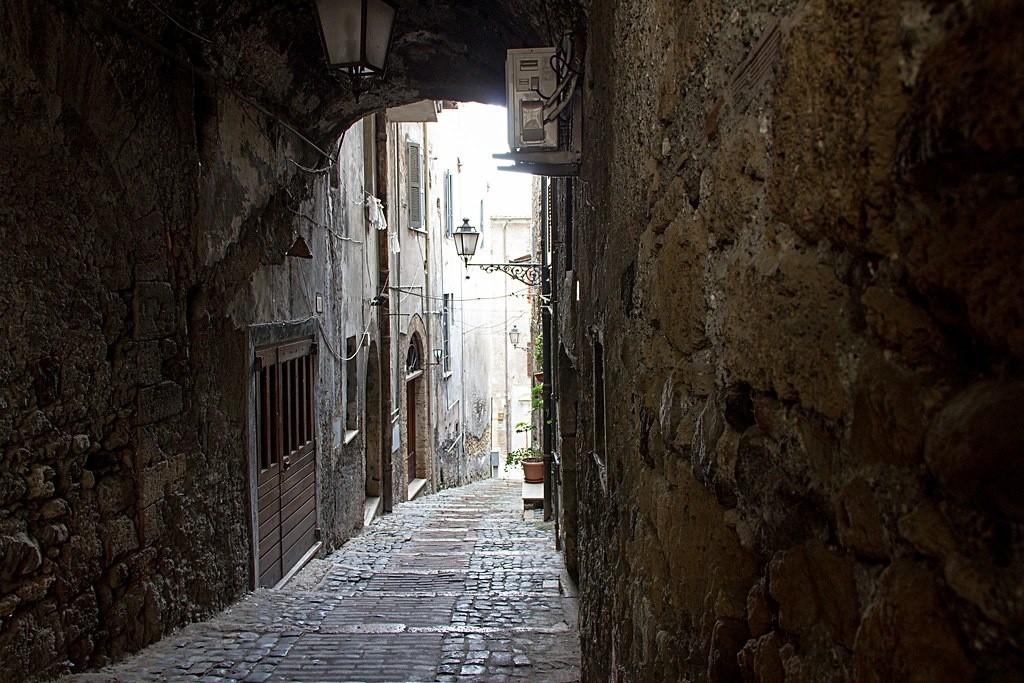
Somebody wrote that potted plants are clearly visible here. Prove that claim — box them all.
[532,331,543,381]
[504,420,545,483]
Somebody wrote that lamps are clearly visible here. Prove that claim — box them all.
[310,0,398,103]
[508,324,531,352]
[452,216,543,286]
[427,345,443,366]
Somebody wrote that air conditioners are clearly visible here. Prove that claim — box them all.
[504,47,561,150]
